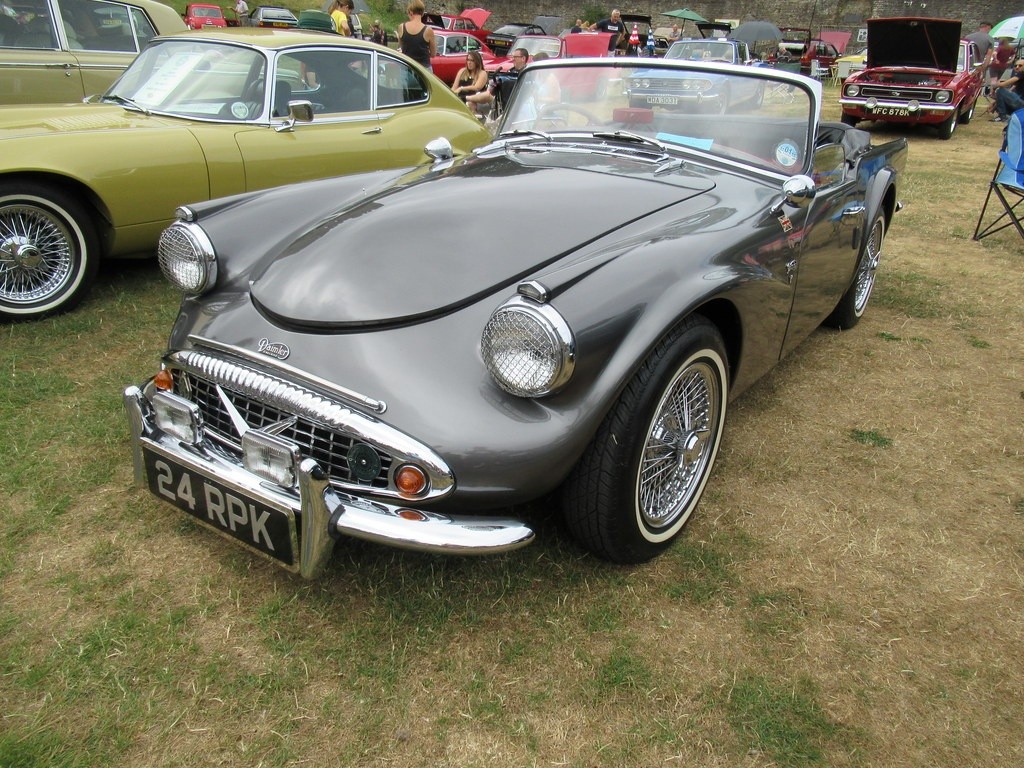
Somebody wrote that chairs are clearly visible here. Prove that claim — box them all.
[973,111,1024,243]
[236,77,291,119]
[763,60,854,104]
[692,49,703,59]
[0,14,20,47]
[14,16,83,49]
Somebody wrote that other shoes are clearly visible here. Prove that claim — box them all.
[993,116,1009,121]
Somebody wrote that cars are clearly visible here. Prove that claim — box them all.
[767,26,868,81]
[621,36,769,113]
[506,34,617,65]
[837,15,986,142]
[429,30,534,89]
[181,3,227,28]
[439,8,493,43]
[484,14,563,53]
[248,5,300,28]
[0,26,494,328]
[618,13,736,57]
[0,0,192,105]
[127,56,910,585]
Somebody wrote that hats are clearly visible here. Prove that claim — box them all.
[980,21,993,29]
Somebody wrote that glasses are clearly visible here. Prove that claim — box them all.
[513,55,523,59]
[466,59,474,62]
[1014,64,1023,68]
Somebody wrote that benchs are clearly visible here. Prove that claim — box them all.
[78,23,147,50]
[603,114,871,163]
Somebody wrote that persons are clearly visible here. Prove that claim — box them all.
[780,47,792,59]
[570,19,590,34]
[372,27,381,43]
[452,51,487,96]
[990,37,1015,97]
[327,0,354,36]
[668,24,681,47]
[965,21,994,67]
[227,0,249,27]
[460,48,529,112]
[398,0,436,73]
[590,9,626,57]
[989,59,1024,121]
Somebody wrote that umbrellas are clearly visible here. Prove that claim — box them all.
[661,8,709,36]
[730,21,783,51]
[988,16,1024,52]
[629,25,639,44]
[646,27,655,50]
